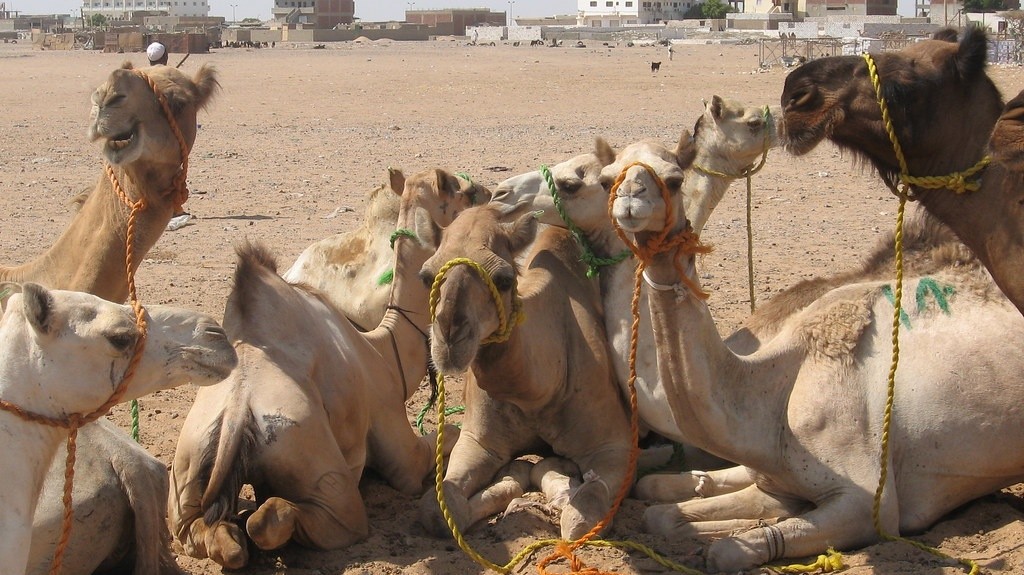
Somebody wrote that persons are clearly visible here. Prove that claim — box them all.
[148,41,168,67]
[668,46,675,61]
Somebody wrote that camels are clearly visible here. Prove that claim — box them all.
[0,16,1024,574]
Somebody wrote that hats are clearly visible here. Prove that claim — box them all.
[147,42,165,62]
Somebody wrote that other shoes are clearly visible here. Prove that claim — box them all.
[173,211,195,220]
